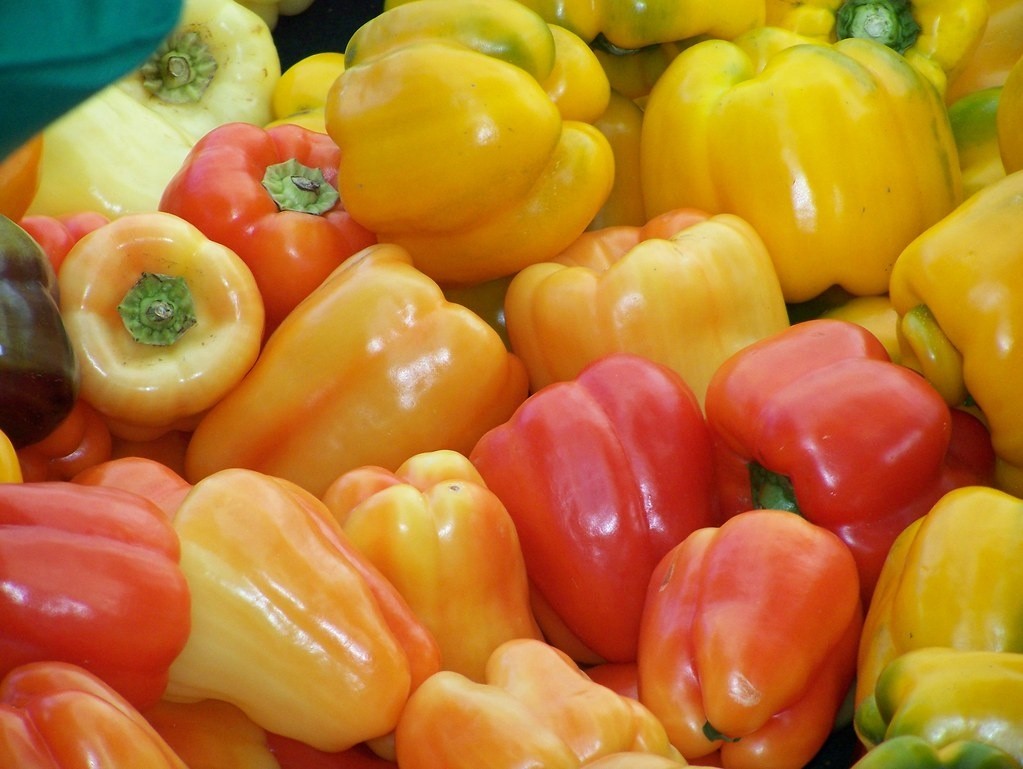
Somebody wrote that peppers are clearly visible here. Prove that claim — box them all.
[0,0,1023,769]
